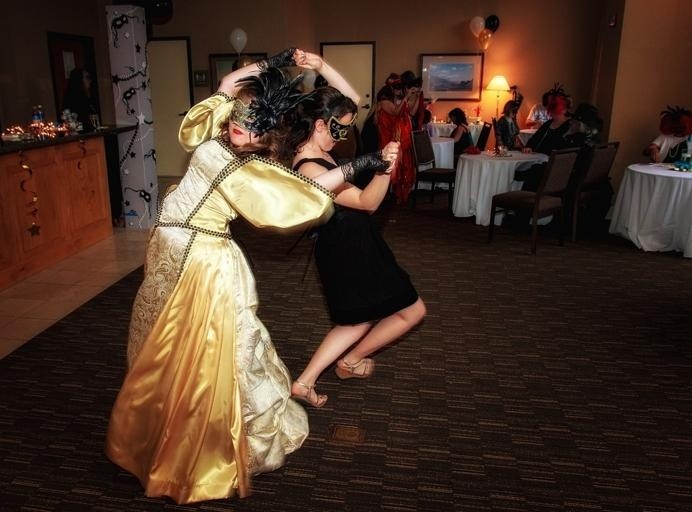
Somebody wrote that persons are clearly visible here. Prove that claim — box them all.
[359,71,427,212]
[494,101,524,150]
[286,49,429,406]
[63,68,100,115]
[522,90,580,156]
[525,98,553,128]
[642,106,691,165]
[514,102,604,231]
[448,108,474,155]
[101,47,398,508]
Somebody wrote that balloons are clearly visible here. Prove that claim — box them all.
[469,15,486,38]
[478,28,494,50]
[485,14,499,32]
[147,1,173,18]
[228,26,248,54]
[237,54,253,69]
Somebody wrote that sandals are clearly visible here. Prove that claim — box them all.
[291,382,328,408]
[334,360,377,380]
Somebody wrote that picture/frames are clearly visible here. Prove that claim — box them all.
[418,52,485,102]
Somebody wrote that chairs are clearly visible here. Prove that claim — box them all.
[351,118,620,255]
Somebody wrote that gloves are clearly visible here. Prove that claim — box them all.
[340,148,391,182]
[255,47,298,72]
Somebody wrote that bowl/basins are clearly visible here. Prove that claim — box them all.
[54,129,66,136]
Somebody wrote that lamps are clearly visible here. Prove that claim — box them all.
[485,73,511,118]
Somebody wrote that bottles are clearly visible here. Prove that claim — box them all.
[31,104,44,123]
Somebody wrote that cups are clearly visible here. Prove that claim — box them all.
[486,143,511,157]
[522,147,532,156]
[432,115,436,123]
[89,114,100,129]
[446,112,453,125]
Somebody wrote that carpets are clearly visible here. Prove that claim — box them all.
[0,189,692,510]
